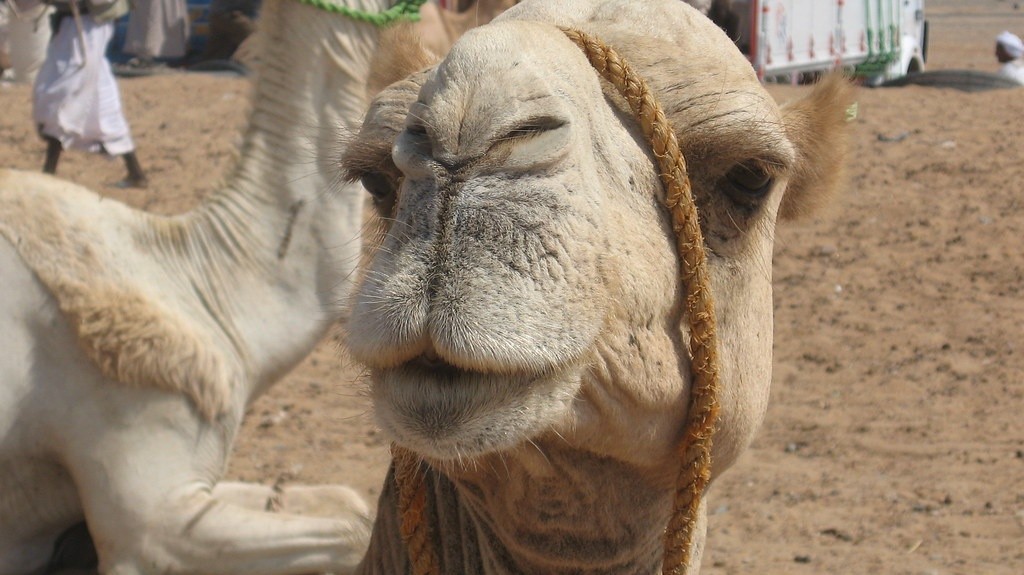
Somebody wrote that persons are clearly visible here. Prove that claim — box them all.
[30,0,149,189]
[995,30,1024,85]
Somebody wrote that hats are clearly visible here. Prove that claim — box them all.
[997,31,1024,59]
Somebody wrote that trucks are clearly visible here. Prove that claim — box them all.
[715,0,930,83]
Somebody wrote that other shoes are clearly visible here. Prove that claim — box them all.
[127,55,153,69]
